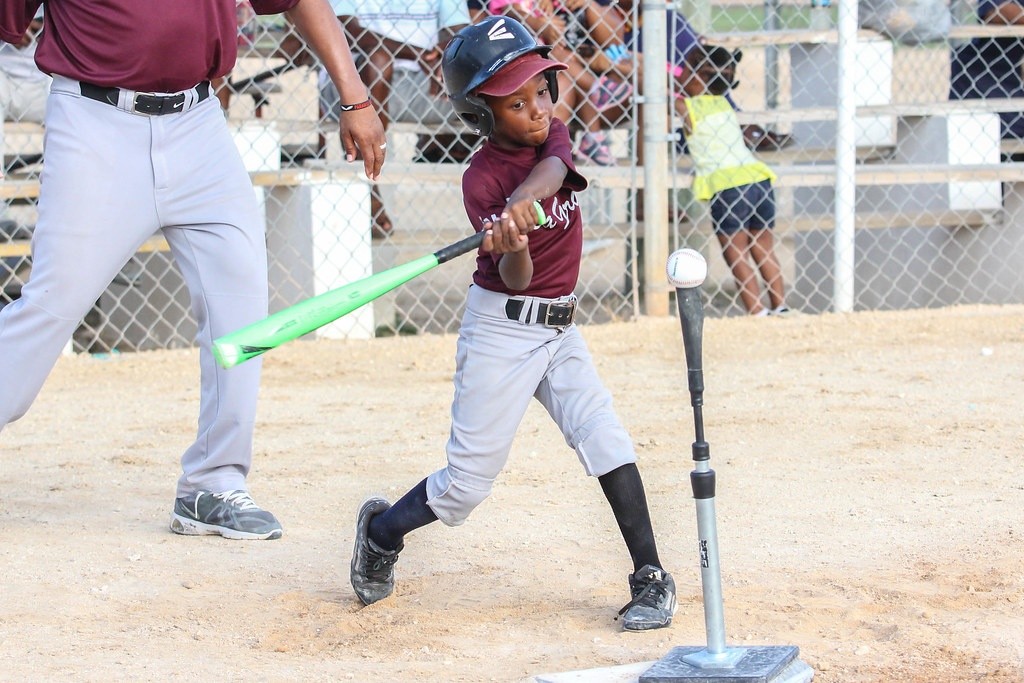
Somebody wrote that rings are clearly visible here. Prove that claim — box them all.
[379,142,386,148]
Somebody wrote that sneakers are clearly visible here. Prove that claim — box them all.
[170,489,284,540]
[574,134,617,165]
[593,73,629,111]
[350,496,405,605]
[614,564,678,632]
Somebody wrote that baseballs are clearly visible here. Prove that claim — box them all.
[665,248,707,288]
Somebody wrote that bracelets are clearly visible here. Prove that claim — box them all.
[340,96,371,110]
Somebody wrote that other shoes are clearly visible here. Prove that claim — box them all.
[748,127,794,149]
[372,194,395,239]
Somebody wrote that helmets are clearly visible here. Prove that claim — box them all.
[442,15,560,136]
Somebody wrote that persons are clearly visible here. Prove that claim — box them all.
[274,0,794,168]
[636,44,786,317]
[0,0,386,538]
[352,14,679,631]
[973,0,1023,84]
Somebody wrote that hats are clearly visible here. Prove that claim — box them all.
[473,51,569,95]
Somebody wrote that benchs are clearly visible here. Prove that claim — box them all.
[0,0,1024,257]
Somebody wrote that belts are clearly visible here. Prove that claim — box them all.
[506,298,578,328]
[80,80,209,116]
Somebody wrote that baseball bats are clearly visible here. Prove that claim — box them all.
[208,200,548,371]
